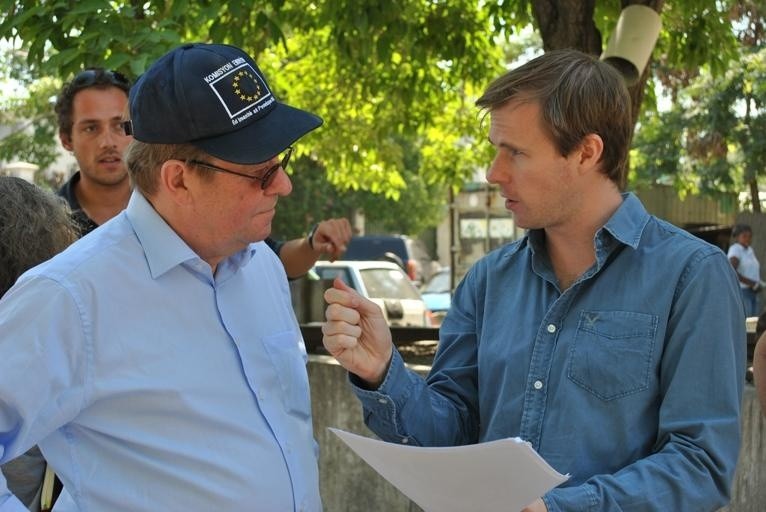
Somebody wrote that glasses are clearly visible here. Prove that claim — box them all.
[73,69,122,87]
[180,146,296,190]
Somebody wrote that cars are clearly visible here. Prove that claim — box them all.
[310,261,450,326]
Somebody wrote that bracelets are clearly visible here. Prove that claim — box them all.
[308,223,319,249]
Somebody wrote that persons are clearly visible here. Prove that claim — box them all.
[1,177,83,511]
[322,50,746,512]
[727,224,766,317]
[55,67,351,282]
[2,43,323,511]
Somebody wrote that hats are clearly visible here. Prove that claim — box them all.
[124,42,324,163]
